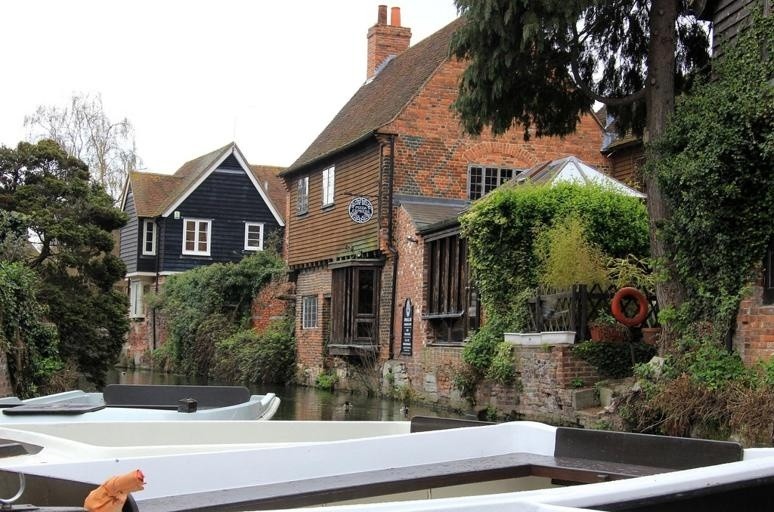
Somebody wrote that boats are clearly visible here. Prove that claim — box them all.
[3,386,282,422]
[3,415,774,511]
